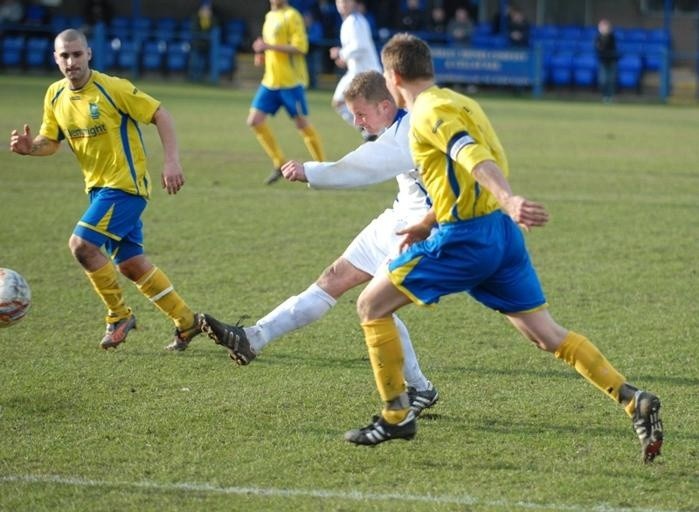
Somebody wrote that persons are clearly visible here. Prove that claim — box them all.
[316,0,530,96]
[1,1,217,60]
[594,19,620,98]
[246,0,326,188]
[342,29,662,465]
[329,0,386,142]
[192,72,440,419]
[9,28,204,353]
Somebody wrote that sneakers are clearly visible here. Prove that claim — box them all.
[200,311,256,366]
[344,380,439,446]
[164,312,202,350]
[262,165,282,186]
[628,389,663,465]
[100,314,137,349]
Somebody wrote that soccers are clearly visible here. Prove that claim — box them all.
[0,267,33,328]
